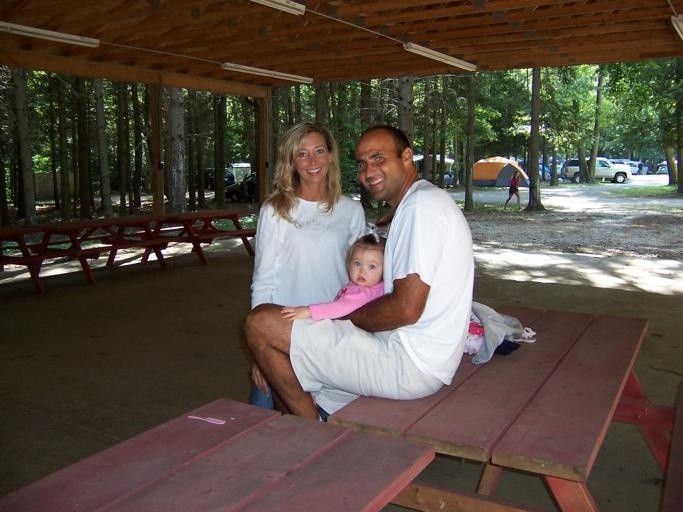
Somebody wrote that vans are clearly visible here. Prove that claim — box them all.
[202,169,234,191]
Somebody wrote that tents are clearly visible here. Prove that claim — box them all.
[518,158,552,182]
[471,155,530,187]
[411,153,459,186]
[655,159,681,174]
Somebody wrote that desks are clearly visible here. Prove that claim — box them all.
[0,208,255,295]
[327,306,683,512]
[0,397,537,512]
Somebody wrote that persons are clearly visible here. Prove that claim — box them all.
[241,124,475,421]
[279,232,386,322]
[503,170,521,211]
[247,121,366,412]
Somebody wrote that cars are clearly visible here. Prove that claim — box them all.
[611,158,643,175]
[655,165,668,174]
[517,158,566,179]
[224,171,258,201]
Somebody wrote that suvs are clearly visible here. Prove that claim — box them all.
[562,156,631,183]
